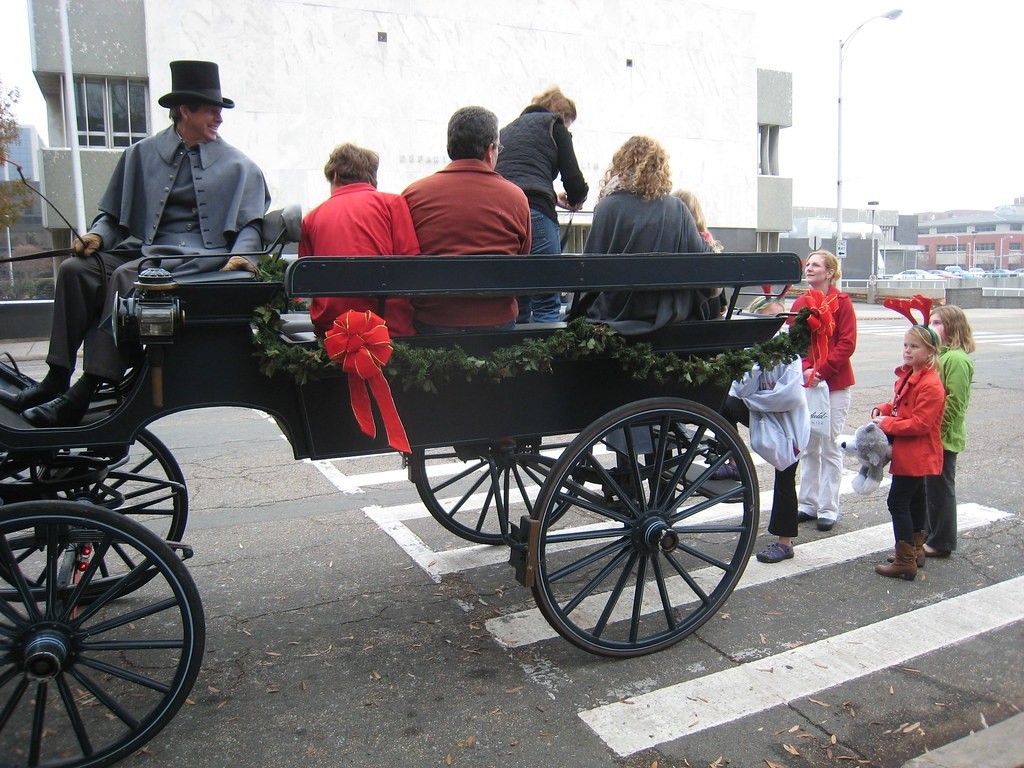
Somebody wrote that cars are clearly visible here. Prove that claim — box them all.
[895,269,934,280]
[928,265,1024,279]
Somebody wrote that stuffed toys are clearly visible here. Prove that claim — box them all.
[835,423,892,495]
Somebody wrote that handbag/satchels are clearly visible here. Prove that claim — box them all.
[806,378,831,440]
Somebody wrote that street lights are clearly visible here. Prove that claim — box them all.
[945,234,958,266]
[1000,236,1014,268]
[867,201,879,275]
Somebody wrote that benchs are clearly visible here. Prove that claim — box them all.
[283,252,803,360]
[132,203,302,327]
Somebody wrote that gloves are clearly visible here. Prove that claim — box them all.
[74,233,101,256]
[220,255,261,279]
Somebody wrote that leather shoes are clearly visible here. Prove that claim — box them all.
[0,384,60,413]
[23,393,83,427]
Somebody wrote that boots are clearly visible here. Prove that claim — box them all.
[887,530,927,567]
[875,540,918,581]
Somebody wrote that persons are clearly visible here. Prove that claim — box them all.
[786,252,857,529]
[709,285,810,563]
[296,142,422,337]
[871,295,946,579]
[669,188,728,320]
[15,60,272,425]
[922,305,976,556]
[402,106,532,334]
[606,422,675,503]
[494,85,589,322]
[560,137,717,336]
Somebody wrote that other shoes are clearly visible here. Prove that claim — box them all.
[921,545,951,557]
[817,518,835,531]
[798,510,816,523]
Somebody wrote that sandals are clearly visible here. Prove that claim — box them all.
[756,540,794,563]
[710,463,741,481]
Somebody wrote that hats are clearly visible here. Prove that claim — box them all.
[158,60,236,108]
